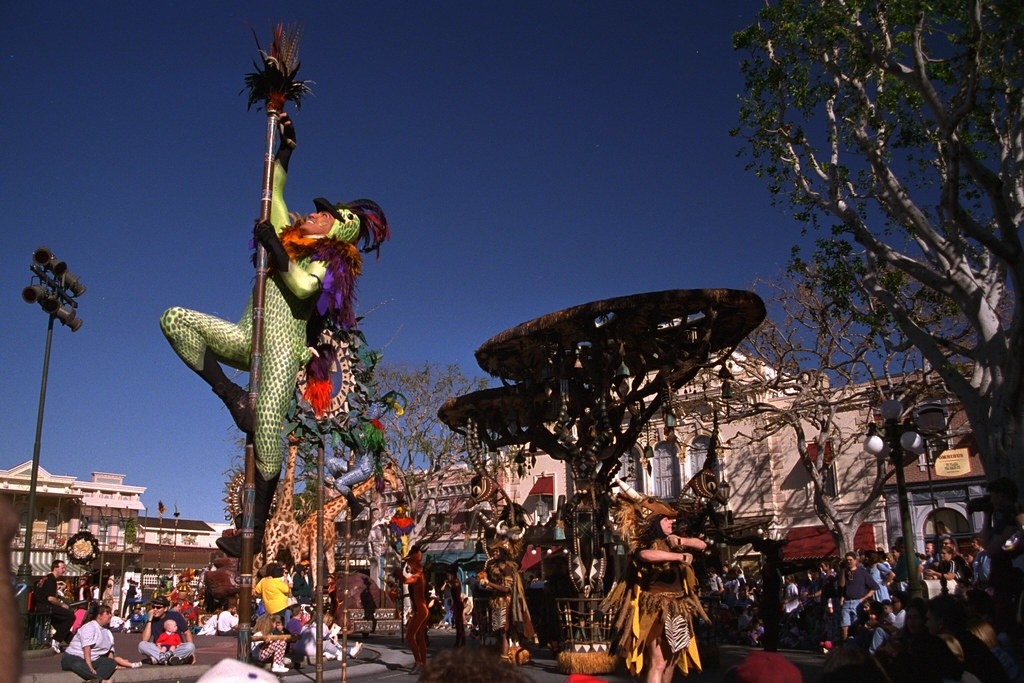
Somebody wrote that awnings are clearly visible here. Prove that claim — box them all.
[807,441,831,463]
[769,523,876,561]
[529,476,553,496]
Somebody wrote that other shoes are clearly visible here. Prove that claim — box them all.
[135,661,142,669]
[159,653,168,662]
[324,653,334,661]
[150,655,158,665]
[169,657,184,665]
[337,652,344,661]
[216,529,262,556]
[211,383,248,425]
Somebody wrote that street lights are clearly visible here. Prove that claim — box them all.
[13,246,86,644]
[863,400,923,600]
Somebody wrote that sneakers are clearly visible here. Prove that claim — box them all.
[272,663,289,673]
[51,638,61,653]
[282,658,291,665]
[350,642,363,658]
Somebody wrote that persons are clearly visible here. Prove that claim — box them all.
[0,106,1024,683]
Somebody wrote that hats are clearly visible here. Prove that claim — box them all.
[152,596,170,606]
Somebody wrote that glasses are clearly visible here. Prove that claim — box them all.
[153,605,166,609]
